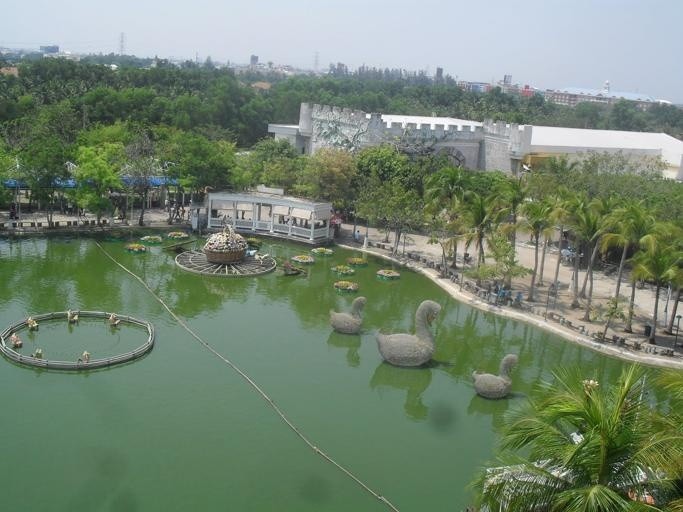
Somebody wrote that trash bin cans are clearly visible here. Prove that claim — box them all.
[644,325,651,336]
[12,223,17,227]
[212,209,217,218]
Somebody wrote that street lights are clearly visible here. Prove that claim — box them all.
[7,158,26,211]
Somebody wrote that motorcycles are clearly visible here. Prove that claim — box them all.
[559,246,583,270]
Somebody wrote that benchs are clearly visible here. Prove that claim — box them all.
[3,217,124,228]
